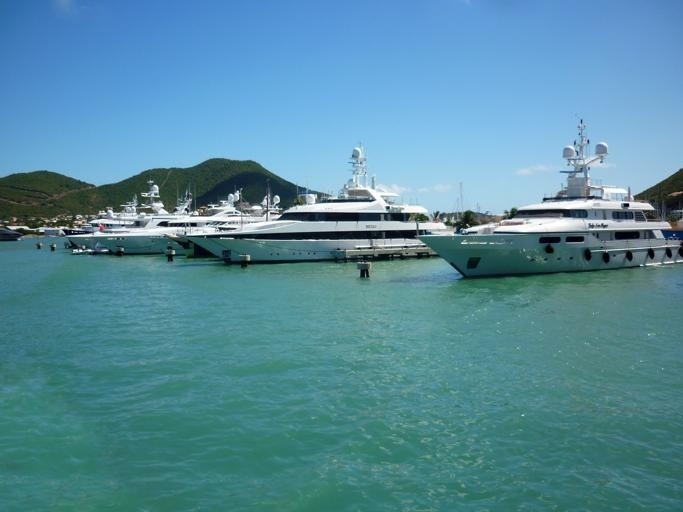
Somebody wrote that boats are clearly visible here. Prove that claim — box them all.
[1,175,244,242]
[176,137,454,265]
[415,118,683,279]
[64,179,317,257]
